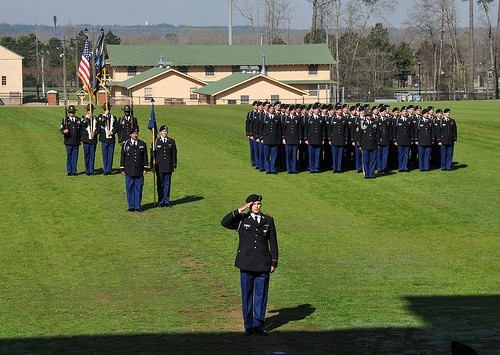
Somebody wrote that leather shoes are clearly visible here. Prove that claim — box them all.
[254,326,268,336]
[244,329,253,335]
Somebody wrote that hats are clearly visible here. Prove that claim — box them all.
[252,100,450,114]
[364,108,372,116]
[129,128,139,134]
[246,194,262,203]
[159,125,168,132]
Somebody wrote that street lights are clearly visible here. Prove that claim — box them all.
[418,59,422,95]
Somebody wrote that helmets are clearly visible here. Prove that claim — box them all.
[85,103,96,109]
[121,105,131,111]
[101,102,112,108]
[66,105,78,111]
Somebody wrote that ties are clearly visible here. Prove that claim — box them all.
[133,141,136,146]
[164,139,165,143]
[270,115,451,124]
[254,216,258,224]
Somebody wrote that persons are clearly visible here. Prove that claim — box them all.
[118,105,139,147]
[120,128,148,212]
[80,103,100,174]
[221,194,278,337]
[60,106,82,176]
[96,102,119,174]
[245,100,457,179]
[149,125,177,207]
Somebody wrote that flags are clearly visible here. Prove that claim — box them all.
[147,104,158,139]
[78,37,108,94]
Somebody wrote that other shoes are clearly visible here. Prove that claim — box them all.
[164,203,169,207]
[104,172,112,175]
[364,177,375,180]
[251,163,451,174]
[136,209,141,212]
[73,173,78,176]
[127,208,135,211]
[66,173,71,176]
[158,204,164,207]
[86,173,93,176]
